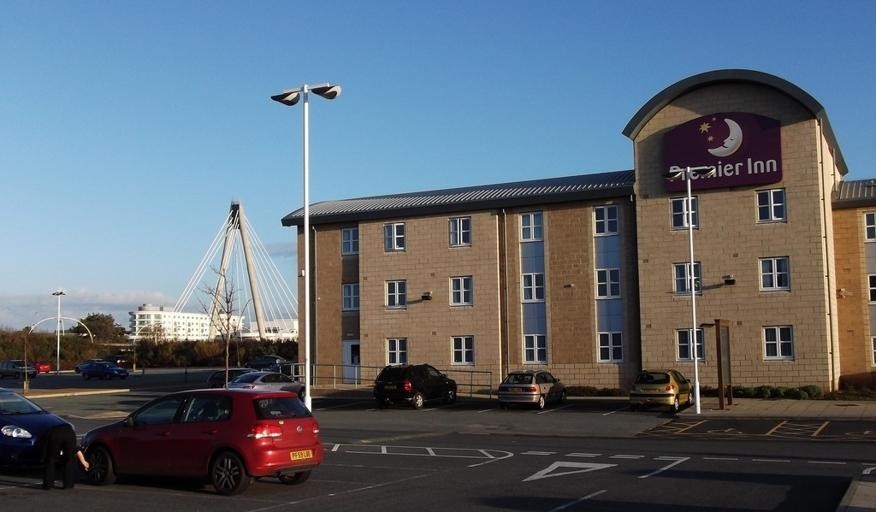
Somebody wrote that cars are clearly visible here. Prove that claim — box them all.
[0,357,39,378]
[625,364,695,413]
[81,361,132,379]
[0,385,82,473]
[71,358,104,374]
[207,368,259,388]
[243,353,287,369]
[496,368,570,410]
[222,370,305,399]
[77,385,329,497]
[32,359,52,374]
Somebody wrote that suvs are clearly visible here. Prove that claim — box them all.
[369,363,459,412]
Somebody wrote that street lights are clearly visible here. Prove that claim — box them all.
[265,79,347,412]
[661,164,721,413]
[51,290,66,374]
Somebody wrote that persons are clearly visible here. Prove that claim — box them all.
[39,423,91,491]
